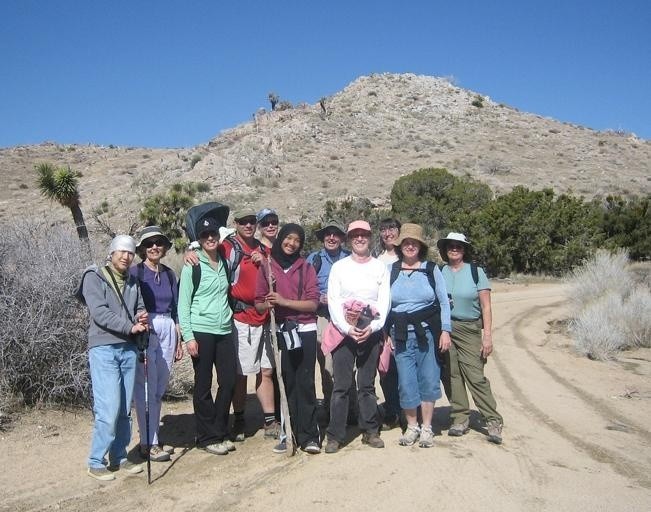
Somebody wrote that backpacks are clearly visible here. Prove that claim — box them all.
[176,255,229,342]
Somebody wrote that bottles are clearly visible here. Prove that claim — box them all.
[283,318,304,352]
[353,304,375,331]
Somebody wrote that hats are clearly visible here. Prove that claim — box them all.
[233,206,259,222]
[135,225,173,260]
[256,207,279,224]
[392,222,430,249]
[347,219,372,237]
[194,215,220,239]
[315,217,348,245]
[436,231,474,253]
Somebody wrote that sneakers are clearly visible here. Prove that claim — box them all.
[231,422,244,441]
[222,441,236,451]
[264,420,282,440]
[419,426,434,447]
[157,443,174,453]
[140,444,170,461]
[399,426,420,445]
[88,468,115,480]
[205,443,228,455]
[448,420,470,436]
[111,460,143,474]
[487,421,503,444]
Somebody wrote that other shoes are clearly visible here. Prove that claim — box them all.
[362,433,384,448]
[381,414,398,429]
[273,439,287,453]
[305,441,321,453]
[325,439,341,453]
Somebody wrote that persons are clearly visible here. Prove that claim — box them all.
[72,235,150,481]
[437,231,504,444]
[129,225,184,461]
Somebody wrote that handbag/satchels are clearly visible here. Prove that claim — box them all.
[276,322,304,354]
[228,295,248,314]
[134,332,148,351]
